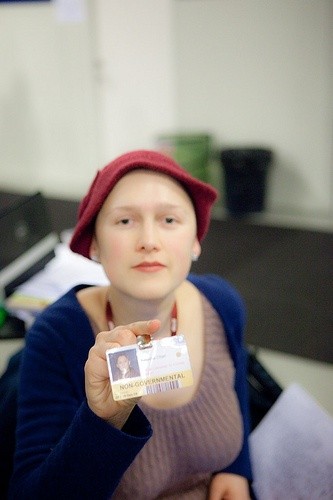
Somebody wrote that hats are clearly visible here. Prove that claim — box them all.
[68,152,219,265]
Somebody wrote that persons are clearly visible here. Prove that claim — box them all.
[13,149,256,500]
[116,355,137,380]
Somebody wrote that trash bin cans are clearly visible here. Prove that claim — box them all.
[222,148,272,213]
[163,132,212,184]
